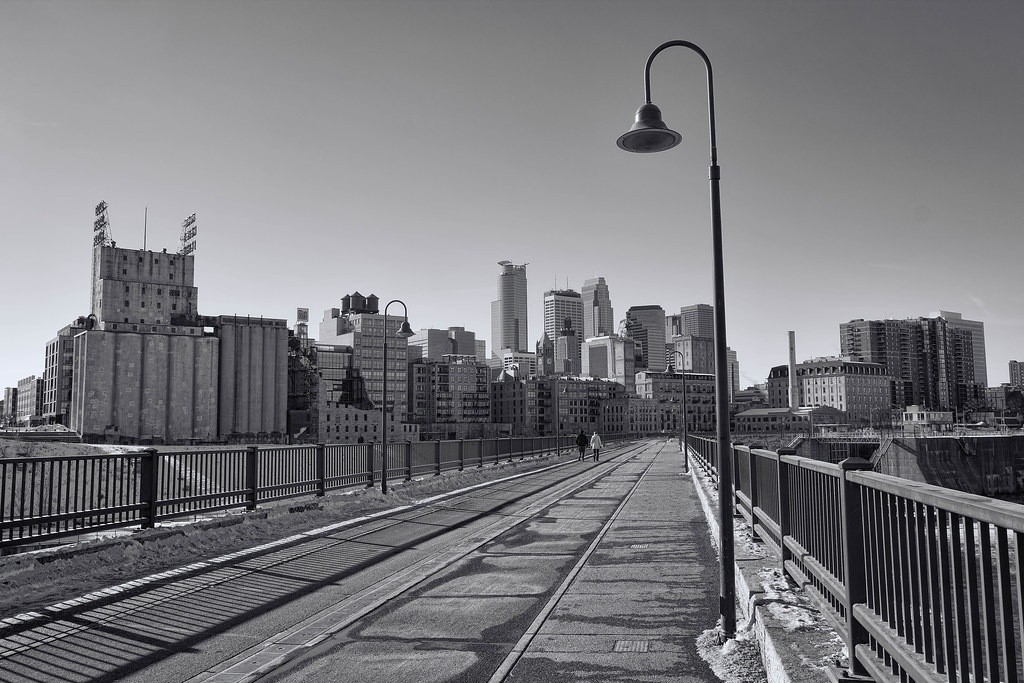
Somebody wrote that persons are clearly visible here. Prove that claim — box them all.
[590,431,602,462]
[575,430,588,461]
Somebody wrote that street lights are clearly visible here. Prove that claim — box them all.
[615,39,737,645]
[665,351,689,473]
[381,300,416,495]
[670,396,682,452]
[556,378,568,457]
